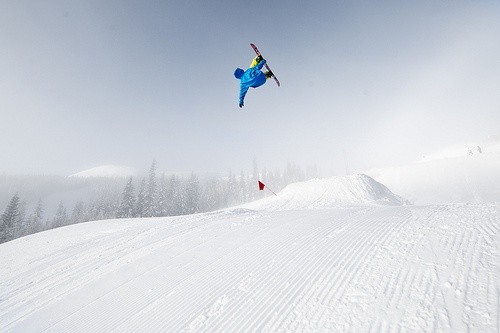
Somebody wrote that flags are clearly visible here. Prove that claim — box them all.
[258,181,265,190]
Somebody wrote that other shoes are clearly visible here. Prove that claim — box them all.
[265,71,273,77]
[255,56,261,63]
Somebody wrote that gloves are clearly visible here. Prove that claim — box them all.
[239,104,244,108]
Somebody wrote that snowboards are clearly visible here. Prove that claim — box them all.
[249,42,281,88]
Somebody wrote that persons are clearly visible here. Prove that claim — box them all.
[234,54,273,108]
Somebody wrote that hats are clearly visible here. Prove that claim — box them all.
[234,68,245,79]
